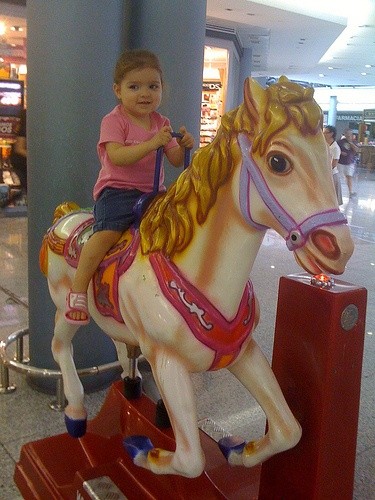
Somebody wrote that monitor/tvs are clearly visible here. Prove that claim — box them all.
[0,79,24,116]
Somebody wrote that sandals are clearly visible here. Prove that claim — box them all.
[65,289,89,324]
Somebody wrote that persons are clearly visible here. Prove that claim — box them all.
[64,50,195,325]
[324,126,369,208]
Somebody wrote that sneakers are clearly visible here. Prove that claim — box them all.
[350,192,357,199]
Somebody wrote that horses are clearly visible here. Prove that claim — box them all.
[39,74,354,480]
